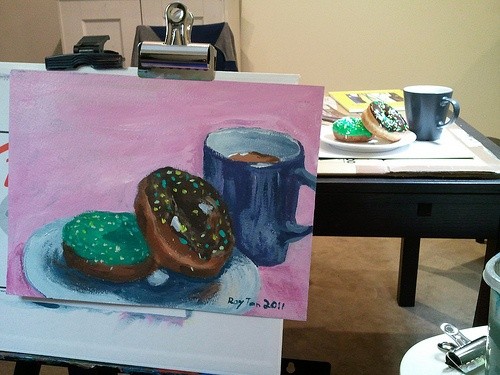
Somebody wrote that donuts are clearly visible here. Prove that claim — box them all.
[332,117,374,142]
[361,100,407,143]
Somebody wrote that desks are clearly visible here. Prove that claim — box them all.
[311,107,500,327]
[399,325,489,375]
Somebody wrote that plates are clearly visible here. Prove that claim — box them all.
[319,125,416,152]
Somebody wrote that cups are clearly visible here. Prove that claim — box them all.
[403,85,460,141]
[483,253,500,375]
